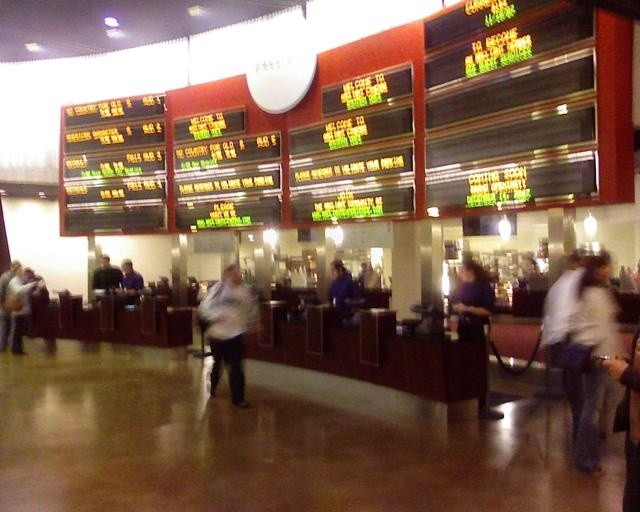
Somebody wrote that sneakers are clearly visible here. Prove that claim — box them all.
[573,460,601,473]
[231,398,248,407]
[478,410,504,420]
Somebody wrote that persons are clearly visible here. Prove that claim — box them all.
[448,256,504,419]
[540,247,640,512]
[92,255,144,305]
[196,263,262,409]
[0,256,57,357]
[327,258,364,317]
[359,262,379,288]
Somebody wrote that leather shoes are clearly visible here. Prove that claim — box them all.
[0,347,28,355]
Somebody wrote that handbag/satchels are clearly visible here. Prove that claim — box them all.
[198,319,208,333]
